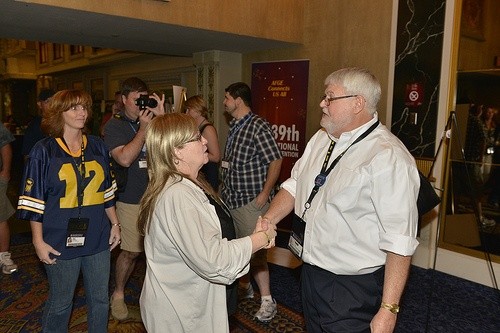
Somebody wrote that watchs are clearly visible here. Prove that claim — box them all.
[381,301,400,314]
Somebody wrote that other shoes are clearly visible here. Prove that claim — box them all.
[110,296,128,320]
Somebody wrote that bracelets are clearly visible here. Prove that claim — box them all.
[258,230,271,247]
[111,222,121,228]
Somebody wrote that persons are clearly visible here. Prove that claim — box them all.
[137,112,280,333]
[253,67,419,333]
[454,99,500,229]
[220,83,284,324]
[182,96,223,194]
[16,87,125,333]
[1,90,19,275]
[99,91,124,136]
[105,78,170,322]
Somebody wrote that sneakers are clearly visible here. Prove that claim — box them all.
[0,252,18,274]
[228,284,254,299]
[253,298,277,324]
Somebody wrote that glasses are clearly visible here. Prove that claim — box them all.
[320,95,366,106]
[186,134,201,143]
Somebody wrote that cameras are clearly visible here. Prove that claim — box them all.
[136,95,158,110]
